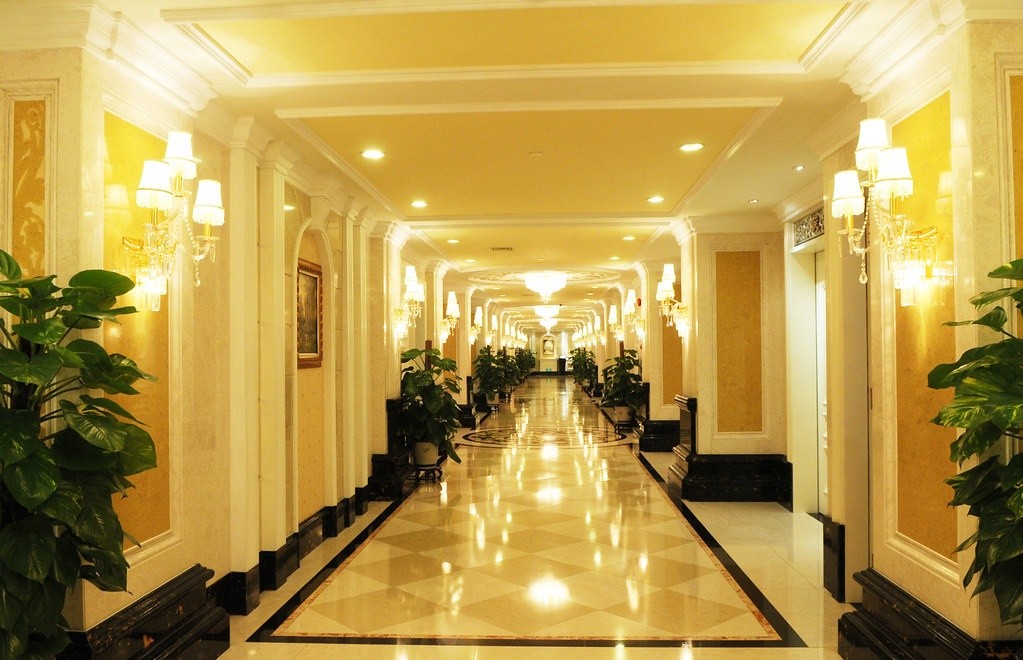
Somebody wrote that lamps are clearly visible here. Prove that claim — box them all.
[124,131,225,311]
[439,291,460,348]
[572,316,606,348]
[469,305,484,346]
[394,264,424,343]
[623,289,645,332]
[656,264,690,327]
[831,118,912,285]
[608,305,624,340]
[486,315,529,349]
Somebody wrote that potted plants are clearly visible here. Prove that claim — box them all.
[598,348,644,421]
[473,346,540,405]
[400,348,463,470]
[569,347,596,386]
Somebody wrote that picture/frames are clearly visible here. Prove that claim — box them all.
[297,258,323,369]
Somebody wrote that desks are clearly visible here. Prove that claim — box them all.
[556,359,566,376]
